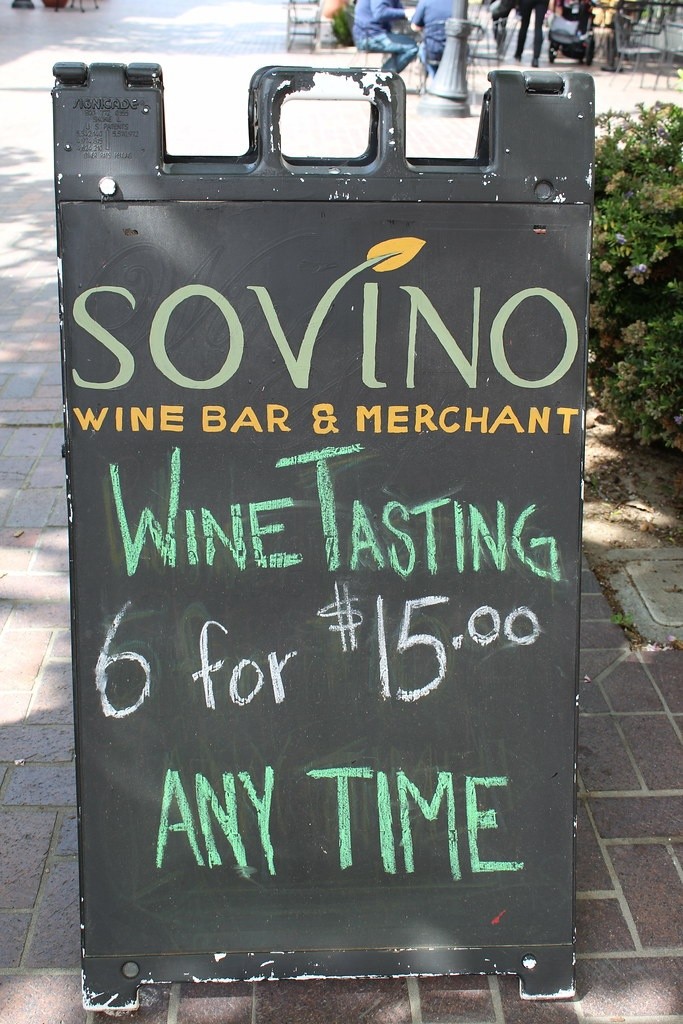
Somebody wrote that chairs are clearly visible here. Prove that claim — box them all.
[286,0,683,107]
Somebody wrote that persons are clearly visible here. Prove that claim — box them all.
[411,0,454,78]
[352,0,419,75]
[489,0,651,73]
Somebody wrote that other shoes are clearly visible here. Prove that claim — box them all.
[532,57,539,67]
[514,52,521,61]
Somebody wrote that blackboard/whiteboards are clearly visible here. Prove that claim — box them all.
[54,72,601,1010]
